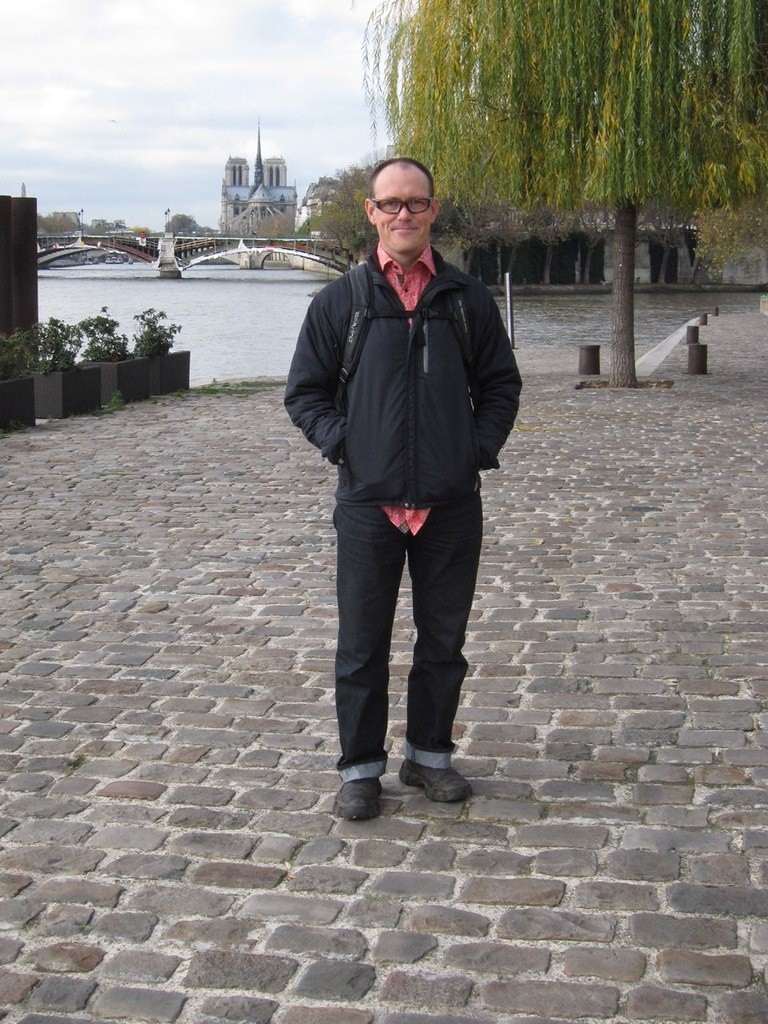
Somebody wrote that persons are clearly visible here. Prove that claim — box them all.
[284,157,523,820]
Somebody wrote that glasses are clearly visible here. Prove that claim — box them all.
[371,198,433,214]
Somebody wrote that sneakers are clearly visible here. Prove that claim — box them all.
[333,777,382,819]
[399,757,470,802]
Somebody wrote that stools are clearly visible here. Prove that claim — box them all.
[687,326,698,345]
[712,306,719,316]
[699,312,707,325]
[688,344,708,375]
[577,343,603,376]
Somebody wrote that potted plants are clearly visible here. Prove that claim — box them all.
[0,326,46,433]
[74,306,151,406]
[132,308,190,396]
[23,317,102,420]
